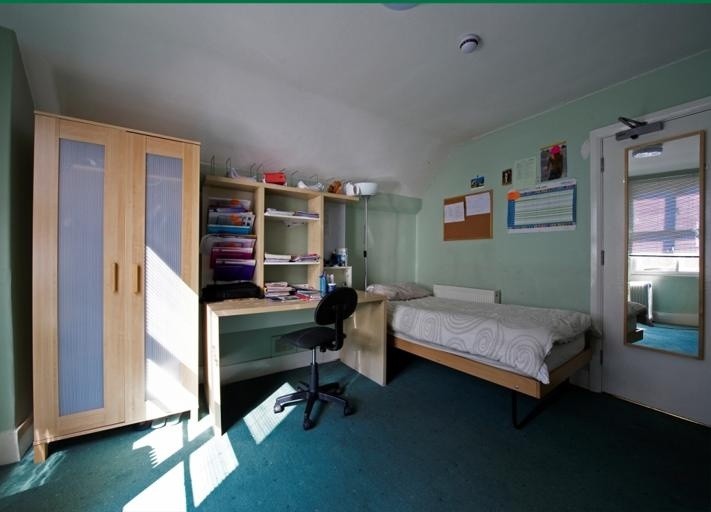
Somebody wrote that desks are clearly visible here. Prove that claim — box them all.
[203,289,388,435]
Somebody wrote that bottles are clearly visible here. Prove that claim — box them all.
[316,270,337,300]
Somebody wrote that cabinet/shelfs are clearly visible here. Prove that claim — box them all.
[30,110,203,464]
[202,175,360,295]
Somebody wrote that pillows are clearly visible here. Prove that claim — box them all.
[365,283,433,300]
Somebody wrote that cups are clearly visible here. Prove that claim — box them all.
[344,180,360,196]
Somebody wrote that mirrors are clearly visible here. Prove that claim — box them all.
[622,130,706,362]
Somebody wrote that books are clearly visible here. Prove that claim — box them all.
[201,196,324,304]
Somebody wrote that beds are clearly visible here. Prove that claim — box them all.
[386,296,593,429]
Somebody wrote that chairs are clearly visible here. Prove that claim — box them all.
[273,287,358,430]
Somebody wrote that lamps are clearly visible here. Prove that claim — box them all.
[355,182,378,291]
[631,143,663,160]
